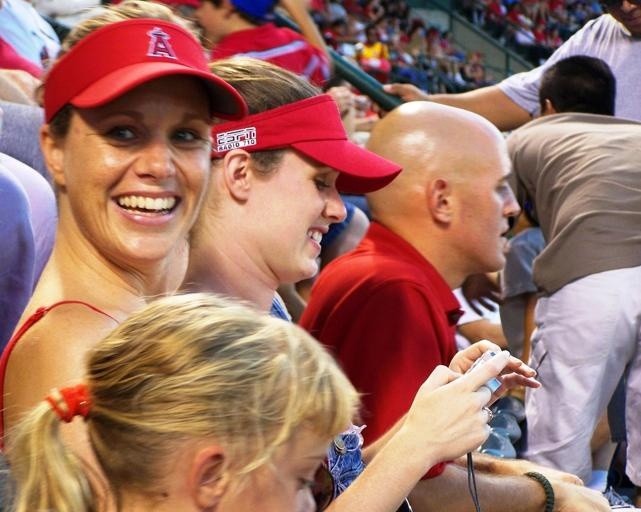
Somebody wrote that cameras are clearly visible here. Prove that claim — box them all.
[463,348,509,410]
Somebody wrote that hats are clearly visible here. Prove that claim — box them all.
[44,18,249,126]
[211,95,403,196]
[233,0,280,17]
